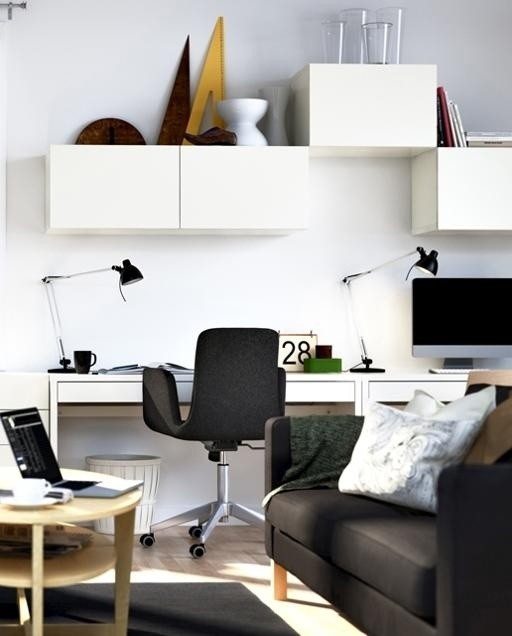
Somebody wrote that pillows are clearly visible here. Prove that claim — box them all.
[280,410,363,491]
[406,386,496,462]
[334,396,483,516]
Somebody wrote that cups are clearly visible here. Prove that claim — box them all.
[335,5,368,64]
[371,5,405,61]
[10,478,51,500]
[358,20,397,64]
[73,350,97,374]
[318,19,345,64]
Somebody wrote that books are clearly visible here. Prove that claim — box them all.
[98,361,194,374]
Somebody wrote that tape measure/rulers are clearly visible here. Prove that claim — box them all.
[182,17,226,145]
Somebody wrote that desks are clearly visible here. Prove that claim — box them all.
[0,372,510,541]
[2,462,141,635]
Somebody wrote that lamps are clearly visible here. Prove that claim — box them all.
[340,244,444,373]
[42,257,151,374]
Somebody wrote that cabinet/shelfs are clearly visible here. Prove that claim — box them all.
[46,144,309,233]
[409,146,510,236]
[288,58,438,148]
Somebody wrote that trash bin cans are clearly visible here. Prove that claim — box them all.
[86,454,162,535]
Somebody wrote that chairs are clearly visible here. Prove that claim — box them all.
[141,328,292,559]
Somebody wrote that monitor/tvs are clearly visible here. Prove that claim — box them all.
[412,278,512,369]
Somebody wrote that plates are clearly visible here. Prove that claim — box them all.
[2,496,57,508]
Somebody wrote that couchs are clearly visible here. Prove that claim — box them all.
[265,383,510,634]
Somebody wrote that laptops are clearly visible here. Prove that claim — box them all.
[0,406,144,498]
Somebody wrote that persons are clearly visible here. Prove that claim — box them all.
[437,85,512,146]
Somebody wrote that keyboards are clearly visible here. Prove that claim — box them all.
[429,369,490,374]
